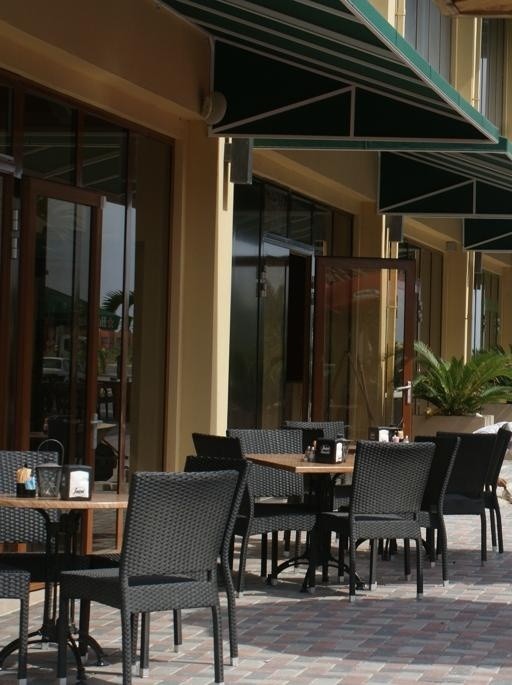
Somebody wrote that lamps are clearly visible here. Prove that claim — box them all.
[36,440,64,499]
[224,138,256,186]
[385,216,404,243]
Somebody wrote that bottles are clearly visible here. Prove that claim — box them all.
[305,446,315,461]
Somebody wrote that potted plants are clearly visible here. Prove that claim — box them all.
[411,338,510,438]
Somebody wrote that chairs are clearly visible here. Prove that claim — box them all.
[2,568,33,685]
[131,456,252,678]
[58,472,235,685]
[191,432,317,594]
[279,420,510,567]
[323,442,437,602]
[227,426,307,580]
[2,447,73,637]
[350,437,458,588]
